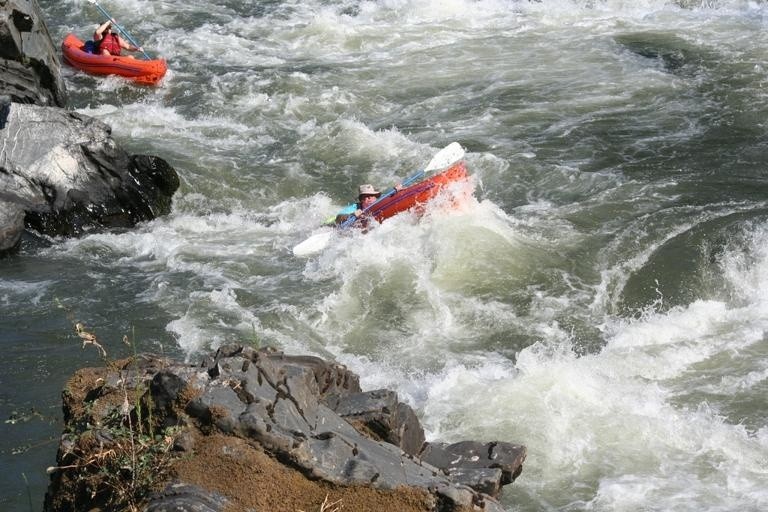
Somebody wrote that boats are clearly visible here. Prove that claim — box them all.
[323,163,467,235]
[62,32,168,84]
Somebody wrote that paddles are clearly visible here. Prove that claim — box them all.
[293,141,465,260]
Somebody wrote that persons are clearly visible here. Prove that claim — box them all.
[94,18,147,60]
[85,29,121,54]
[316,183,407,228]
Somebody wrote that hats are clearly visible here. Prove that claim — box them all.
[358,183,382,202]
